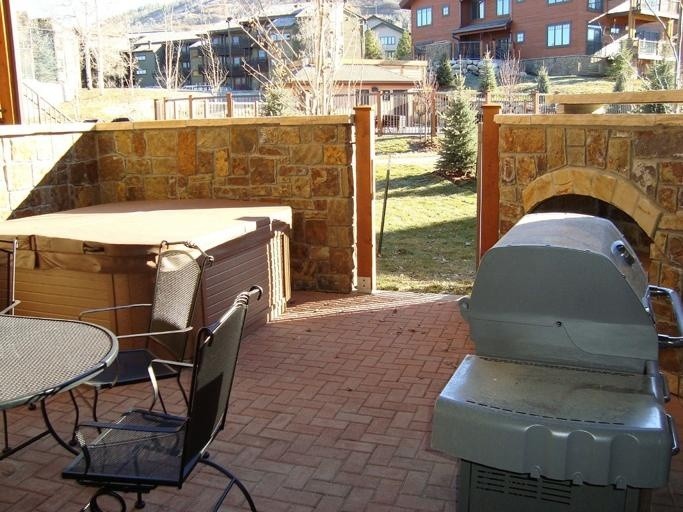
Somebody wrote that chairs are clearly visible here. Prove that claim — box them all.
[0,240,262,512]
